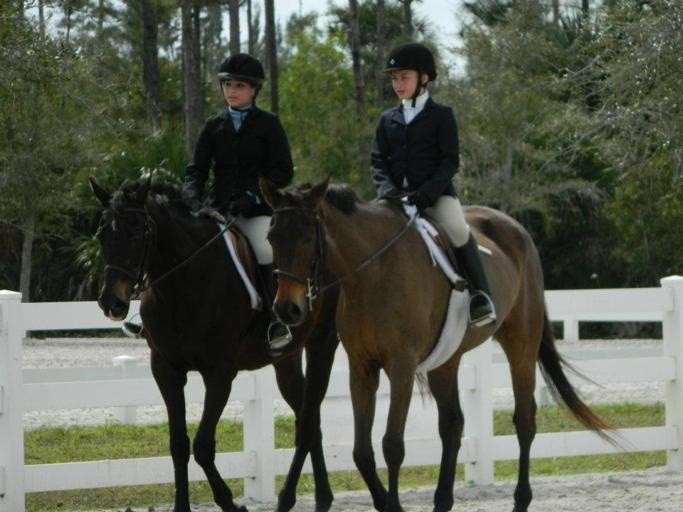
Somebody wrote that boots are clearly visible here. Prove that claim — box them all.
[453,230,491,316]
[258,261,288,340]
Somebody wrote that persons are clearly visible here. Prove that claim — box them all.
[366,44,498,327]
[120,52,293,360]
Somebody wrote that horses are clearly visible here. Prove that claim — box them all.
[88,171,339,512]
[258,171,641,512]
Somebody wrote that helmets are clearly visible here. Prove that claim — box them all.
[218,53,264,85]
[384,43,437,81]
